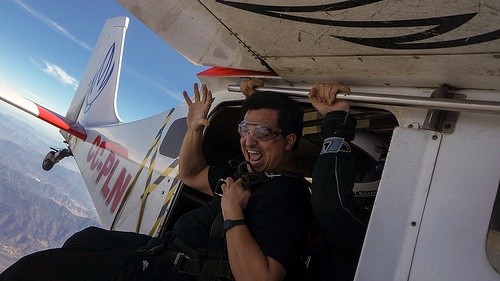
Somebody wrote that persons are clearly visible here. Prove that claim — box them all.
[241,76,387,281]
[0,81,310,281]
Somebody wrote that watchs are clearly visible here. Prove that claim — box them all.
[223,218,247,235]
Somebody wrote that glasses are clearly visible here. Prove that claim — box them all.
[238,120,285,140]
